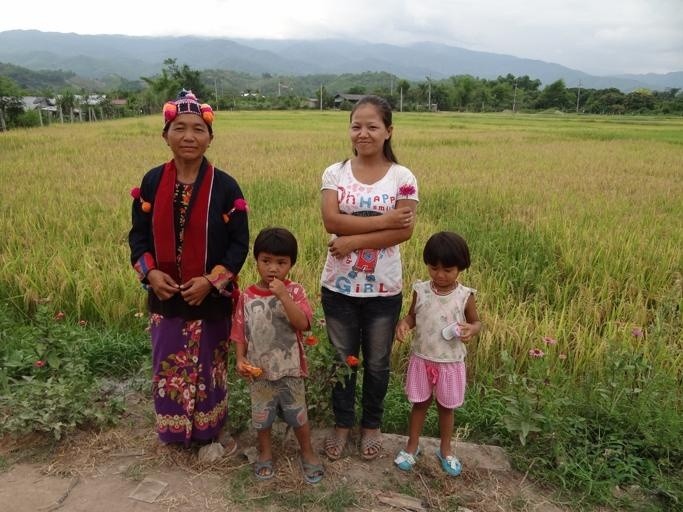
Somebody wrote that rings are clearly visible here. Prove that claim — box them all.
[405,218,409,223]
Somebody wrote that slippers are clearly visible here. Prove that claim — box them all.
[296,450,326,485]
[323,428,350,460]
[393,441,423,473]
[358,424,384,461]
[435,445,464,477]
[251,454,277,480]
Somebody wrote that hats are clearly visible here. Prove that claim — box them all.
[159,85,217,131]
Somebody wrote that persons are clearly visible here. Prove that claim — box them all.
[128,101,249,464]
[392,231,481,476]
[319,93,419,461]
[230,228,325,484]
